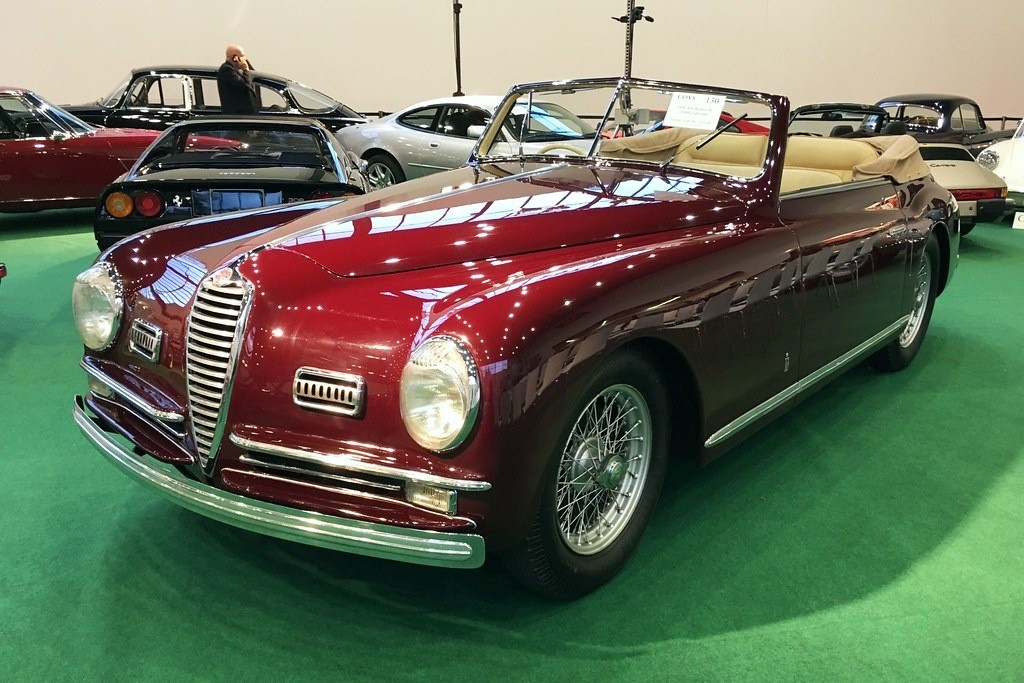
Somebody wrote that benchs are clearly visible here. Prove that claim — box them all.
[673,134,879,193]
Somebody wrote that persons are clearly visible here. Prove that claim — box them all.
[216,45,263,116]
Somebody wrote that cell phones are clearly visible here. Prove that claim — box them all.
[232,55,240,64]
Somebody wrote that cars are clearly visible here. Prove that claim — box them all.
[596,108,769,140]
[92,112,371,256]
[69,80,961,604]
[976,116,1024,221]
[334,90,611,195]
[55,64,372,127]
[858,88,994,143]
[1,89,250,220]
[783,100,1007,239]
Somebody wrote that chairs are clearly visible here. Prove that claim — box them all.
[884,122,907,136]
[830,125,854,138]
[450,112,471,136]
